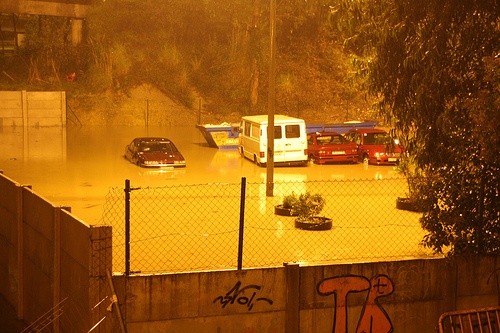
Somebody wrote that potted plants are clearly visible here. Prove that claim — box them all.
[274,192,333,230]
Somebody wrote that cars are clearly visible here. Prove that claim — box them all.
[125,137,186,168]
[308,132,360,164]
[343,128,404,163]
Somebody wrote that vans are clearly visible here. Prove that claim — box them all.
[238,114,309,166]
